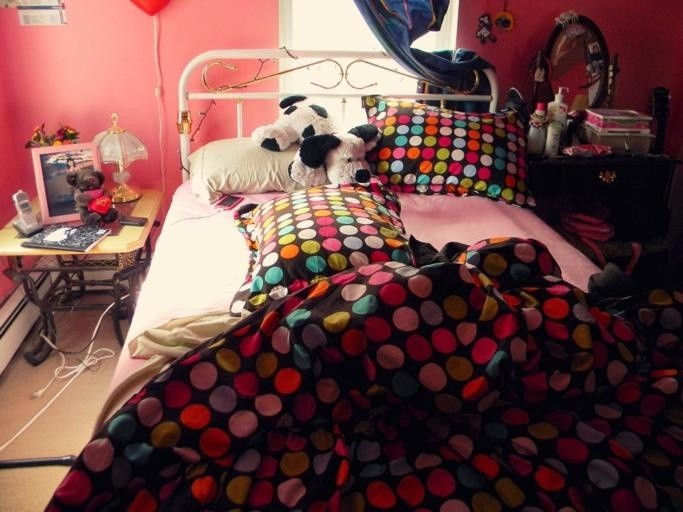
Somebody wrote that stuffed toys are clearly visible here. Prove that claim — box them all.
[250,93,379,187]
[66,169,119,224]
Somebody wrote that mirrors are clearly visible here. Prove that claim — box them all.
[544,14,609,122]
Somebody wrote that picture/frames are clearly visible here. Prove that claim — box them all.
[29,142,103,227]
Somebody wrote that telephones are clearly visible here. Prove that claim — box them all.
[10,189,44,238]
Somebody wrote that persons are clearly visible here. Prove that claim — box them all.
[66,157,83,211]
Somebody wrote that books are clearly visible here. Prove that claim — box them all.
[21,223,113,253]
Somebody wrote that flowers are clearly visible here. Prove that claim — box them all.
[24,122,79,148]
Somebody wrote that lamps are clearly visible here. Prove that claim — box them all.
[90,112,148,203]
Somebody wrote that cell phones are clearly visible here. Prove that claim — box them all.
[120,216,148,226]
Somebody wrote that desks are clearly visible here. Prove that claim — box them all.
[525,151,682,244]
[0,190,163,366]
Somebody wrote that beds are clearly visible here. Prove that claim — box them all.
[95,47,604,439]
